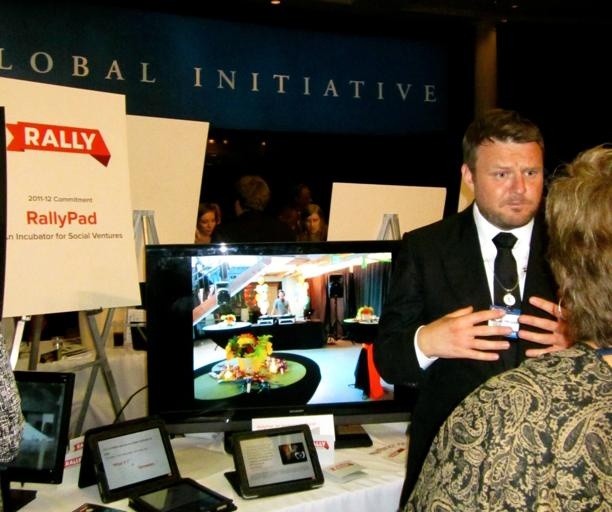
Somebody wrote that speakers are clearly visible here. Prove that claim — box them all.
[327,274,344,298]
[213,281,230,306]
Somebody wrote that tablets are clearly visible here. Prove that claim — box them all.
[77,413,181,504]
[128,478,234,512]
[223,423,324,500]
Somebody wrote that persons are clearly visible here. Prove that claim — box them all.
[372,106,573,511]
[270,289,291,315]
[194,171,329,247]
[399,139,612,510]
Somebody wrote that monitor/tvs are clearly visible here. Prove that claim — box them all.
[143,238,419,453]
[304,308,314,319]
[0,369,76,512]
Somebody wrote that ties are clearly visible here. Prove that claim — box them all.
[491,232,520,373]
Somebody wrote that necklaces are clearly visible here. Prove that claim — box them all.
[480,253,529,307]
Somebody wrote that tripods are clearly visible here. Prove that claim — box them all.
[331,298,356,345]
